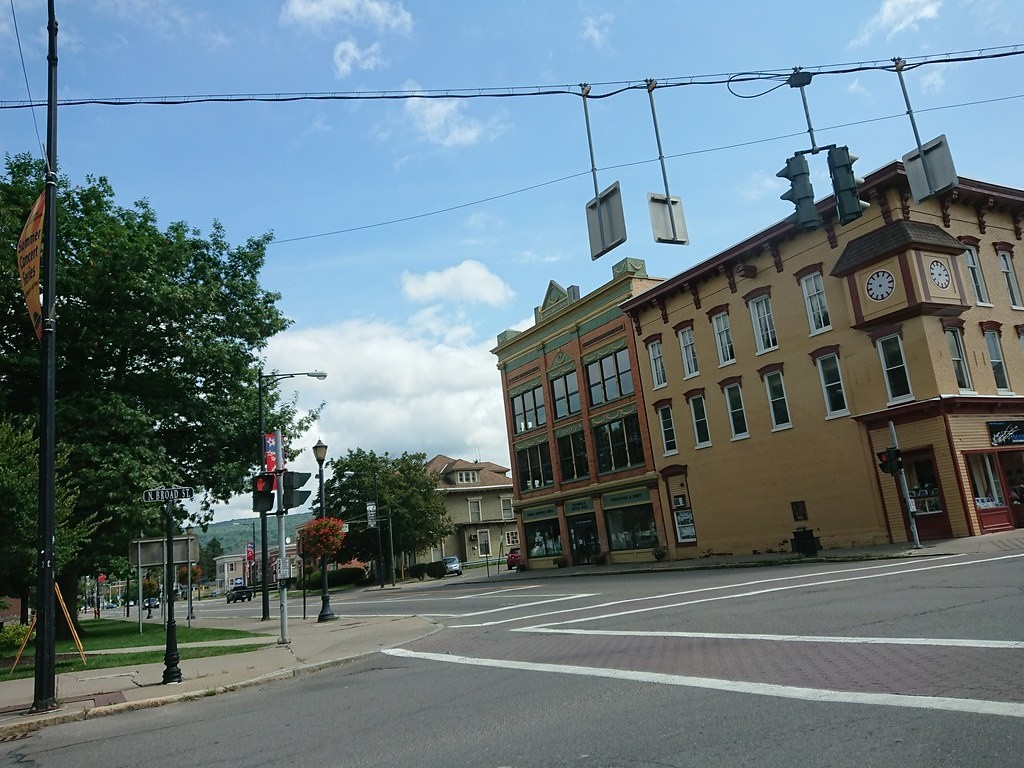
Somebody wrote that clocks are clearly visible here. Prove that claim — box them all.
[929,260,952,290]
[865,269,896,302]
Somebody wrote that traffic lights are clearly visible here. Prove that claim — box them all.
[891,447,905,473]
[249,475,276,512]
[876,451,890,474]
[832,147,874,224]
[773,149,821,230]
[283,471,311,510]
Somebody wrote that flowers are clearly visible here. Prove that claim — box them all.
[297,515,348,567]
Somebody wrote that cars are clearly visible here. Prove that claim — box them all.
[442,555,462,576]
[504,547,523,570]
[143,598,160,609]
[227,586,252,604]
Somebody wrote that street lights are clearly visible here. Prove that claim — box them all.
[258,367,328,622]
[185,524,196,620]
[233,522,256,598]
[311,437,336,624]
[344,471,385,589]
